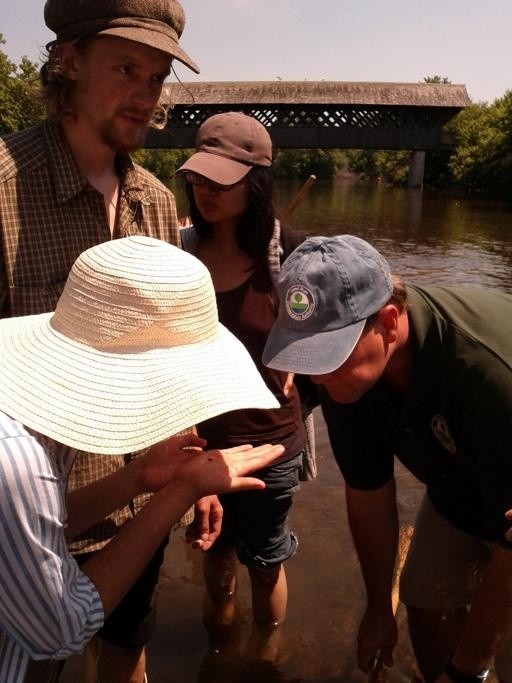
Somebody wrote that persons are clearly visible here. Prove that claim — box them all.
[1,0,205,682]
[171,108,332,680]
[0,236,287,681]
[262,232,512,682]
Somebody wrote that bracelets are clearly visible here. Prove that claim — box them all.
[442,653,491,682]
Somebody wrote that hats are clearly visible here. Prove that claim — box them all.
[173,112,272,187]
[44,1,201,74]
[262,235,394,376]
[0,236,282,455]
